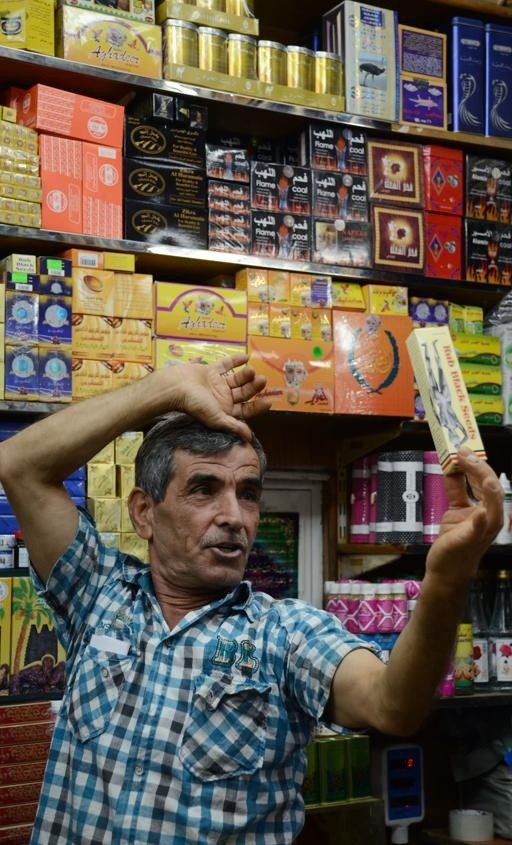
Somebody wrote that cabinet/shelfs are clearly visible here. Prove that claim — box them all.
[1,45,511,290]
[2,397,512,703]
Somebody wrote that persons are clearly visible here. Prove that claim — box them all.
[1,354,504,844]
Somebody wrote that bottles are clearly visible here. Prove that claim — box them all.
[467,567,512,694]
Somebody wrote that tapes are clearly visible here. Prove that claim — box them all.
[448,809,495,842]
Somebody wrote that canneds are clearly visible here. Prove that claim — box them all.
[160,1,344,99]
